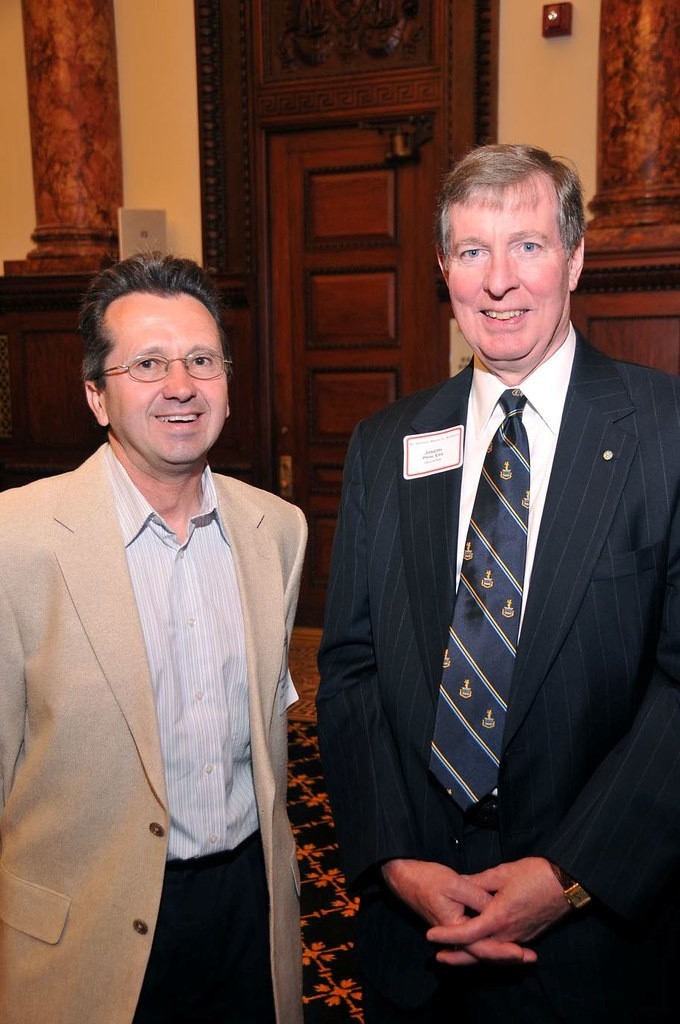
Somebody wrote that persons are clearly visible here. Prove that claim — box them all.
[0,253,308,1024]
[316,142,680,1024]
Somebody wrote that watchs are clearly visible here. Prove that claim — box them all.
[549,861,591,909]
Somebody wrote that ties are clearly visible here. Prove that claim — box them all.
[428,389,531,809]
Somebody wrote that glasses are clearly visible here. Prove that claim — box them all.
[101,351,232,380]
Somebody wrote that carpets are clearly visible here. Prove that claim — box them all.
[288,714,365,1024]
